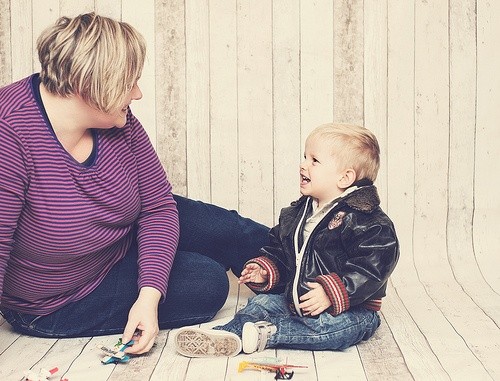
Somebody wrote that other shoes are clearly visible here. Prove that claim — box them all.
[174,326,243,358]
[242,321,277,354]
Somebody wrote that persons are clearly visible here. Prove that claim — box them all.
[0,12,285,355]
[174,123,399,357]
